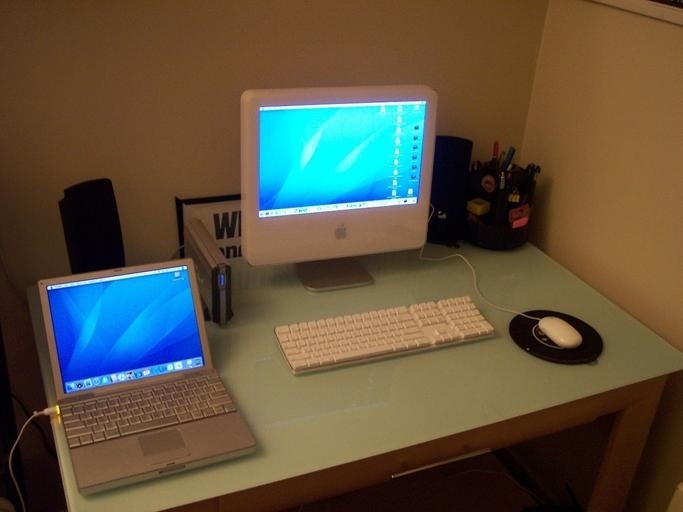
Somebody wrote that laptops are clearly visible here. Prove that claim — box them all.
[37,258,258,496]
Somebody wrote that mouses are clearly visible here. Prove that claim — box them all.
[538,316,582,349]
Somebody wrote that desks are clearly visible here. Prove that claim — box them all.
[26,216,683,512]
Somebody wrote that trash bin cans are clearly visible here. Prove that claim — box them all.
[393,469,536,512]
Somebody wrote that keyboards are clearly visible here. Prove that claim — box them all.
[273,294,494,377]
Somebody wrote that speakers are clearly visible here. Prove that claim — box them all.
[427,135,473,248]
[58,177,125,275]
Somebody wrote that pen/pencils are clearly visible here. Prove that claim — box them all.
[471,141,540,192]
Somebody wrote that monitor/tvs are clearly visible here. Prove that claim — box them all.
[239,86,438,293]
